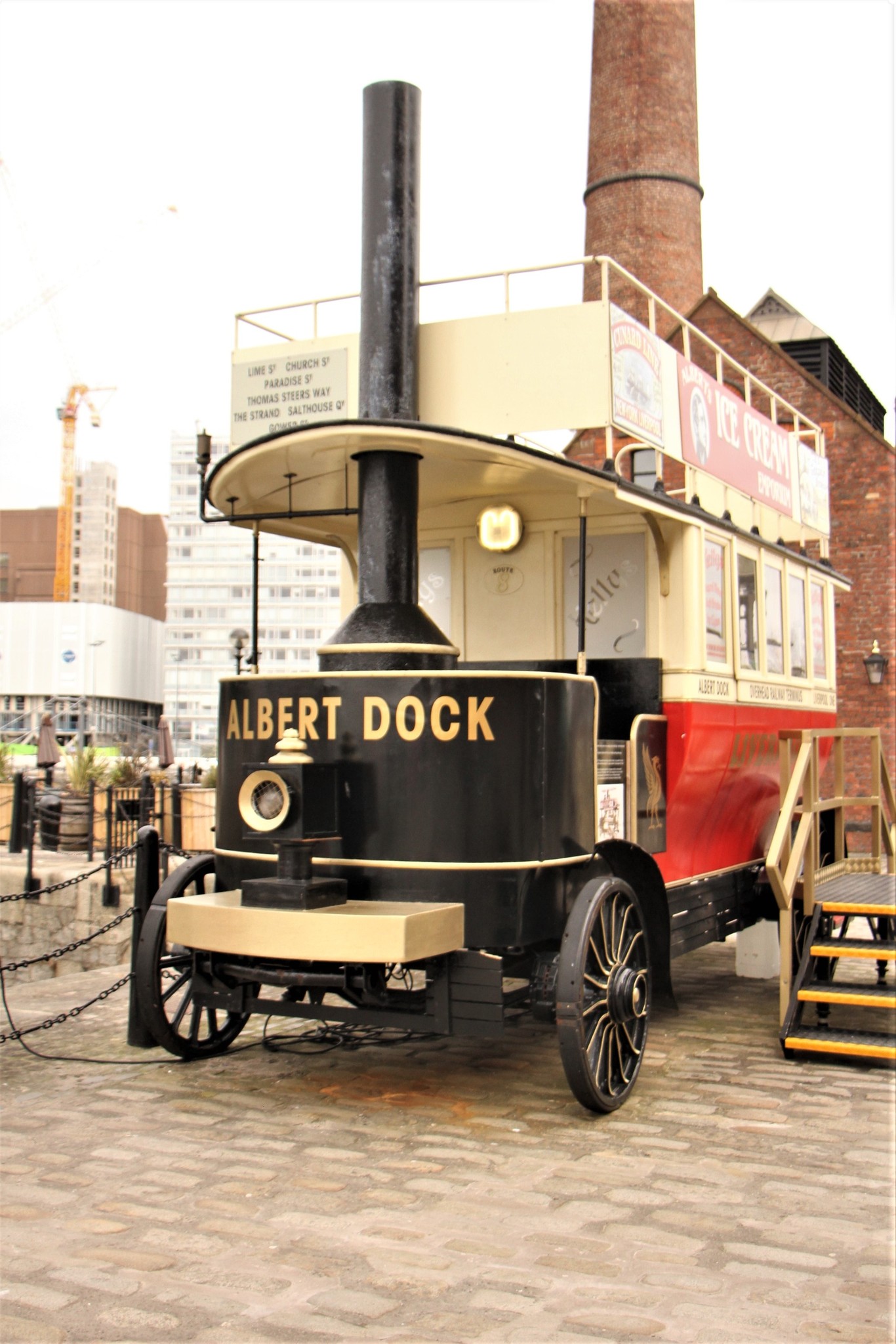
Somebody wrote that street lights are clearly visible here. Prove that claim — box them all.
[231,628,248,676]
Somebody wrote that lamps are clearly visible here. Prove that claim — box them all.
[863,639,888,686]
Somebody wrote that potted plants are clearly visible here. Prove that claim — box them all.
[0,735,217,852]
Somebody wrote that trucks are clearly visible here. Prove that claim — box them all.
[138,83,857,1114]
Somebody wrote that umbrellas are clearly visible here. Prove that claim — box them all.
[156,716,174,775]
[36,715,60,786]
